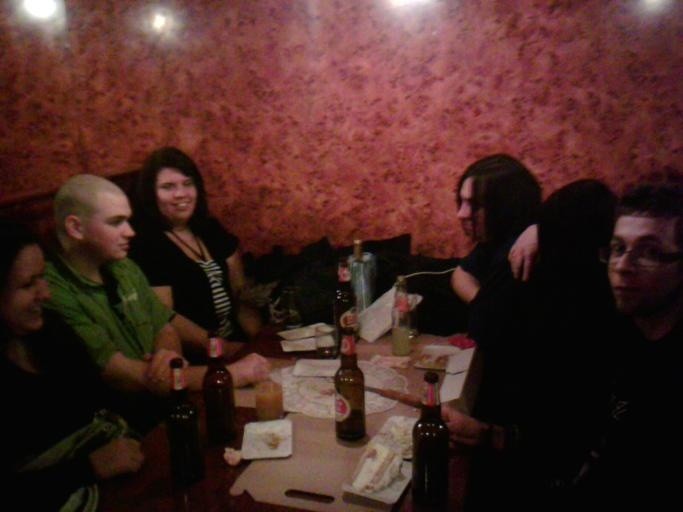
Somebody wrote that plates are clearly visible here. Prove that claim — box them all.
[416,343,456,371]
[243,418,293,460]
[340,416,419,506]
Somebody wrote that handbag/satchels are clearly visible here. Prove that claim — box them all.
[21,408,143,512]
[260,283,354,359]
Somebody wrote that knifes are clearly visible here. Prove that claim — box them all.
[365,386,424,409]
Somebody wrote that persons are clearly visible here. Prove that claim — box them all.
[480,176,615,361]
[419,186,678,507]
[135,145,288,361]
[1,232,145,507]
[35,174,273,395]
[438,147,545,320]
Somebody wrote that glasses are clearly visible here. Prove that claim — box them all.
[597,242,682,267]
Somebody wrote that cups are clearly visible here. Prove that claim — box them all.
[316,326,339,357]
[282,287,304,329]
[253,363,284,422]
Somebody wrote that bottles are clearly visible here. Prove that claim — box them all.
[333,265,362,344]
[392,276,412,355]
[334,327,365,443]
[350,241,372,312]
[167,358,204,483]
[412,373,450,511]
[204,333,236,443]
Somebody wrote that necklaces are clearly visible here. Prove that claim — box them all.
[68,258,107,287]
[166,230,212,265]
